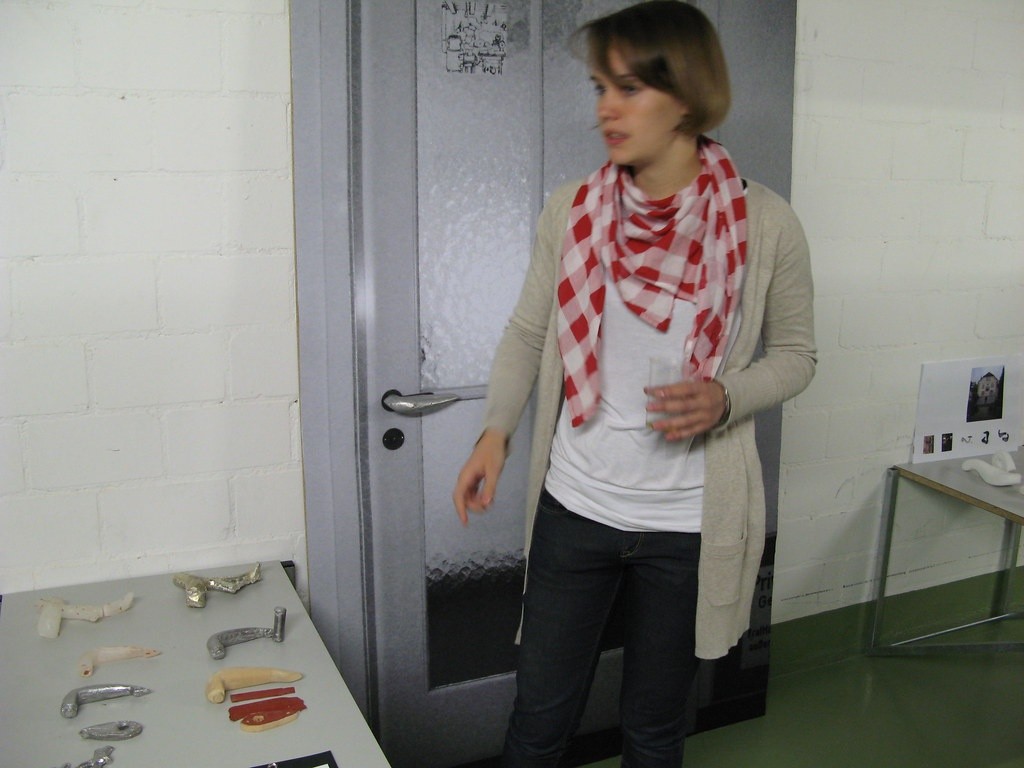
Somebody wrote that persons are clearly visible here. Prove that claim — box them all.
[451,0,819,768]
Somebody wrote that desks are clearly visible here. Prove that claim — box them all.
[866,445,1023,661]
[0,560,392,768]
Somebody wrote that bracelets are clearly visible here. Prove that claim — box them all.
[719,389,730,426]
